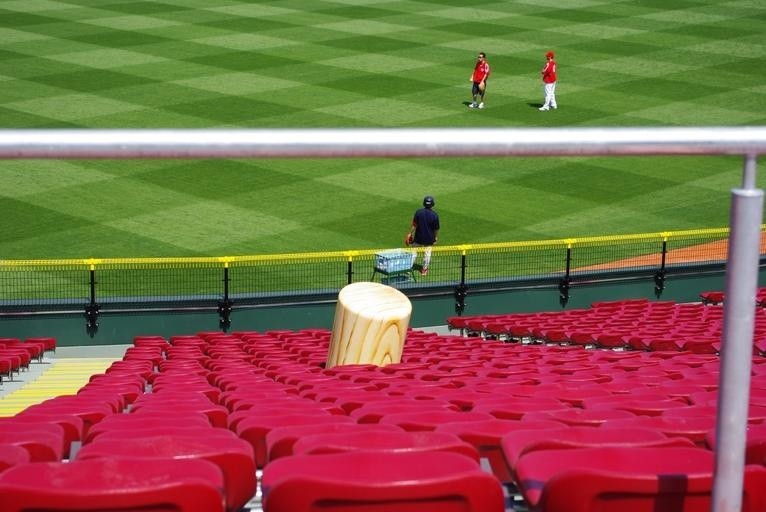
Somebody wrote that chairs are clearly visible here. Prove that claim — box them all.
[0,283,766,512]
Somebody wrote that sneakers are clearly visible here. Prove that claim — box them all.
[538,106,550,111]
[421,268,428,275]
[477,104,484,109]
[552,106,557,109]
[469,103,478,108]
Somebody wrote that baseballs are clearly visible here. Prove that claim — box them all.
[470,79,472,81]
[377,260,399,270]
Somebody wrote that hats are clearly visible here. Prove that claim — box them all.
[545,52,553,57]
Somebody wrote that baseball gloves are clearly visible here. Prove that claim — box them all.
[405,233,414,246]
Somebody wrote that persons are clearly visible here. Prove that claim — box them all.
[539,52,558,110]
[468,51,489,109]
[406,197,441,275]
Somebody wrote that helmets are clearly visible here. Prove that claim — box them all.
[423,196,434,208]
